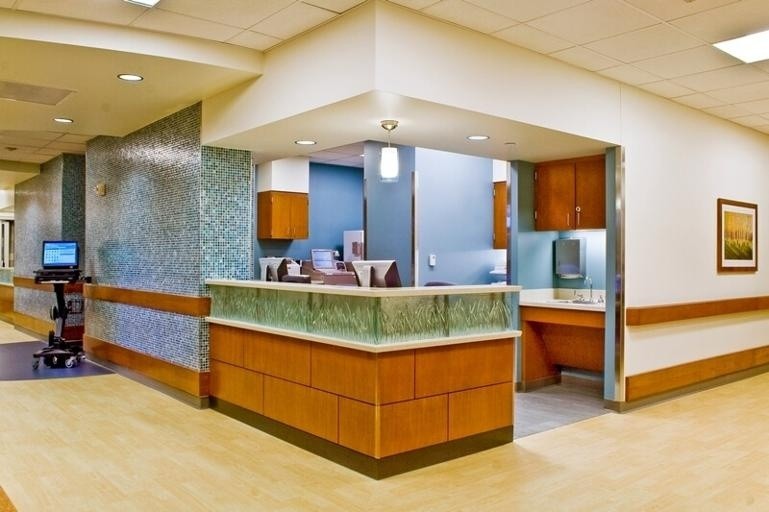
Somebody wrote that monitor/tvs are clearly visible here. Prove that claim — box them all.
[311,248,336,270]
[42,240,78,267]
[259,257,288,281]
[352,260,402,287]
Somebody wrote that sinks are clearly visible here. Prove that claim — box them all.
[549,300,598,304]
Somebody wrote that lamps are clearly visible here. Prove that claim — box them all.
[378,119,399,178]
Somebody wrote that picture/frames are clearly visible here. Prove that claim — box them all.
[717,197,759,271]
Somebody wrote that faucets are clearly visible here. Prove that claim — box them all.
[583,277,593,300]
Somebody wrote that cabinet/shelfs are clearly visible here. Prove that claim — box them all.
[534,153,606,232]
[257,190,309,240]
